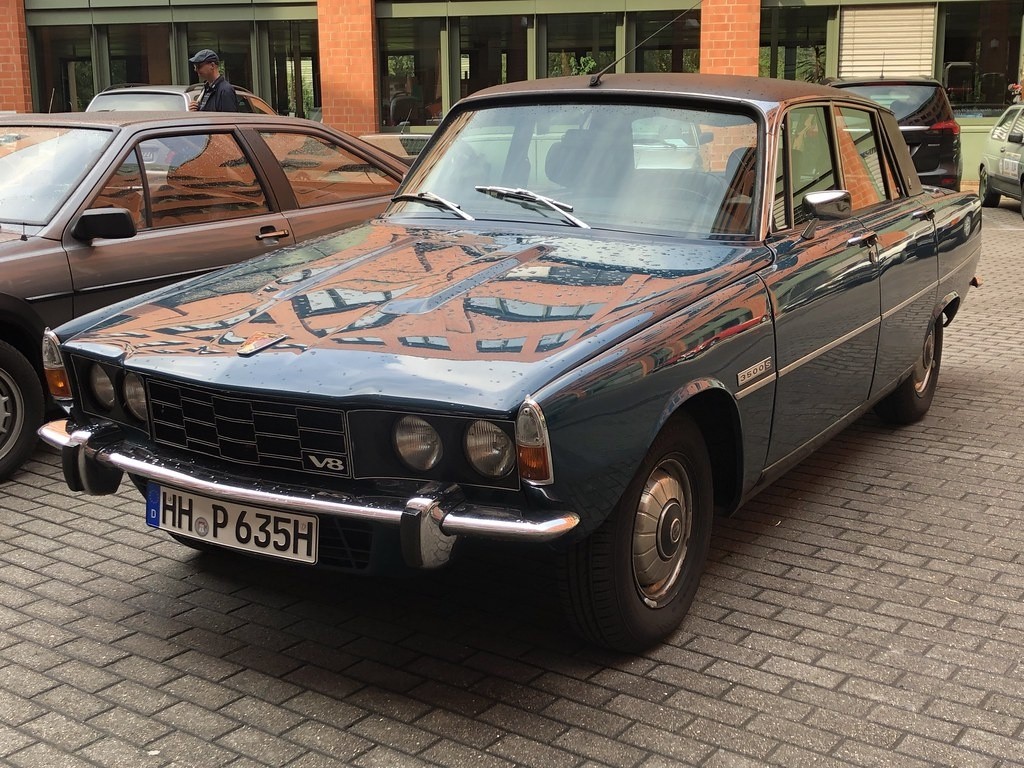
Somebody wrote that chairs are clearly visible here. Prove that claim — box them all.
[543,133,625,217]
[700,145,802,236]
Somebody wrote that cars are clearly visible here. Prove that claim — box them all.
[566,109,714,170]
[976,104,1024,221]
[34,69,987,656]
[1,110,415,481]
[787,73,964,195]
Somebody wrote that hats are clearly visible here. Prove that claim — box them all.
[188,49,220,62]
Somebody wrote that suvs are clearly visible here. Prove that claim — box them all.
[84,82,284,177]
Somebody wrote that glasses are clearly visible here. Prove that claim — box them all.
[193,63,207,71]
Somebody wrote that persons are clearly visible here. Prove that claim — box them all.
[188,49,240,112]
[411,70,436,126]
